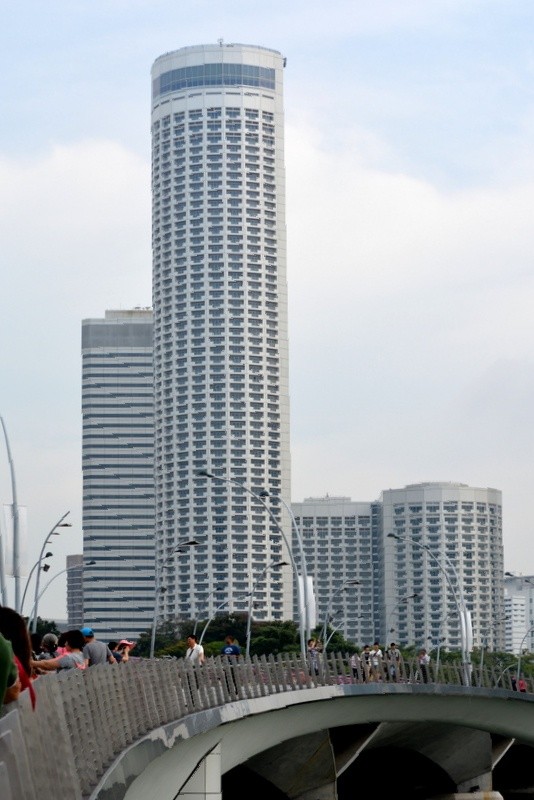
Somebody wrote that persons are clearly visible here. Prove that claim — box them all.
[184,635,205,707]
[511,676,526,693]
[419,649,431,684]
[221,636,240,695]
[350,642,401,681]
[307,638,322,676]
[0,607,134,716]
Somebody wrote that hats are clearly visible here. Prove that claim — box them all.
[40,633,58,648]
[119,639,133,645]
[80,628,93,636]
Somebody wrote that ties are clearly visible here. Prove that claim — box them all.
[189,650,193,657]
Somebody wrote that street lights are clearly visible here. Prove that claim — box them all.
[198,470,311,660]
[151,541,200,655]
[505,572,534,691]
[315,579,360,657]
[388,532,474,686]
[247,561,290,660]
[193,586,251,645]
[21,511,97,634]
